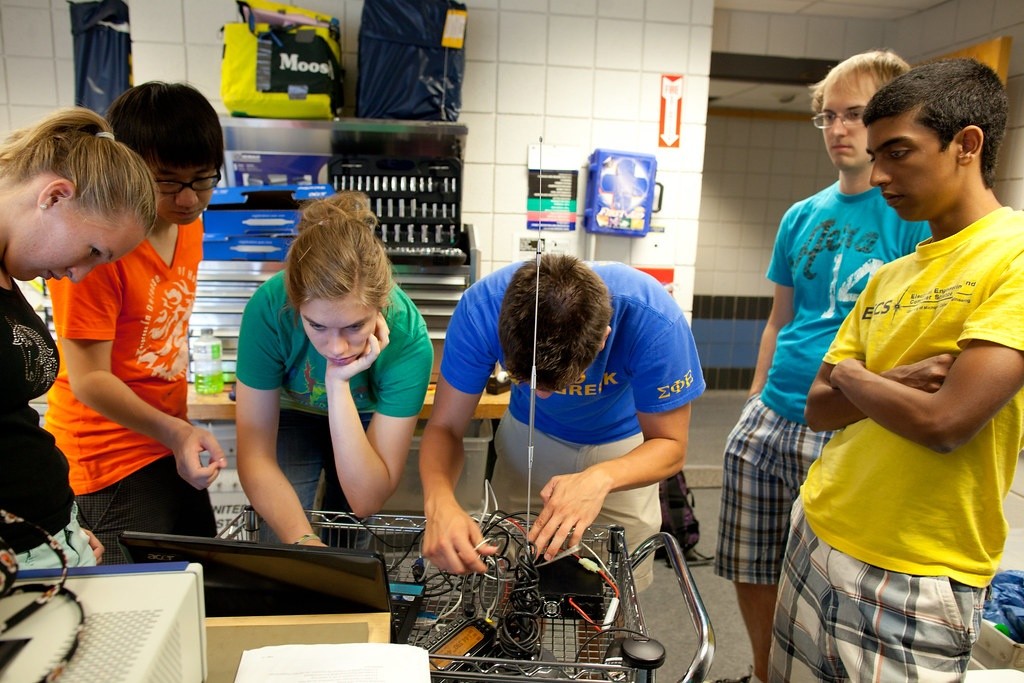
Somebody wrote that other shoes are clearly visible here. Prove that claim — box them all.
[710,663,753,683]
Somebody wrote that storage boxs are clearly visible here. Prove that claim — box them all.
[200,183,335,236]
[215,0,349,120]
[584,149,662,239]
[967,619,1024,669]
[380,419,495,514]
[199,233,297,262]
[327,154,467,264]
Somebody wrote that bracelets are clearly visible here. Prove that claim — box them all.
[294,534,322,542]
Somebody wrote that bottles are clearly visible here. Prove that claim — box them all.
[192,329,222,394]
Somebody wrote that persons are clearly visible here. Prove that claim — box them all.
[236,190,434,554]
[712,51,932,683]
[769,59,1024,683]
[45,82,228,560]
[0,108,160,571]
[419,256,707,593]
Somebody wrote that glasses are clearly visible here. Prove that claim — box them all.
[150,168,221,195]
[811,111,865,130]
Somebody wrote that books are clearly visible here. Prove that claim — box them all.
[189,150,335,383]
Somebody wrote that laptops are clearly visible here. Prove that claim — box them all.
[118,532,427,645]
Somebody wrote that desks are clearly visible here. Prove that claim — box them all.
[202,611,394,683]
[187,381,517,421]
